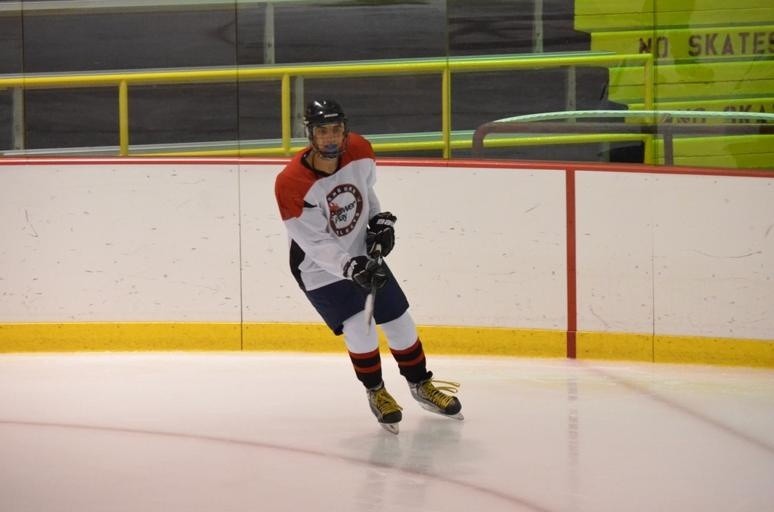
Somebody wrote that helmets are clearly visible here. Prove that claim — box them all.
[303,98,351,158]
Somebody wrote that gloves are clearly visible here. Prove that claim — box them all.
[343,253,389,292]
[365,212,397,259]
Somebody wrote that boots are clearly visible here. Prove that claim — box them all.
[407,375,465,422]
[365,386,403,435]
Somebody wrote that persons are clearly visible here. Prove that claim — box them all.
[274,99,461,421]
